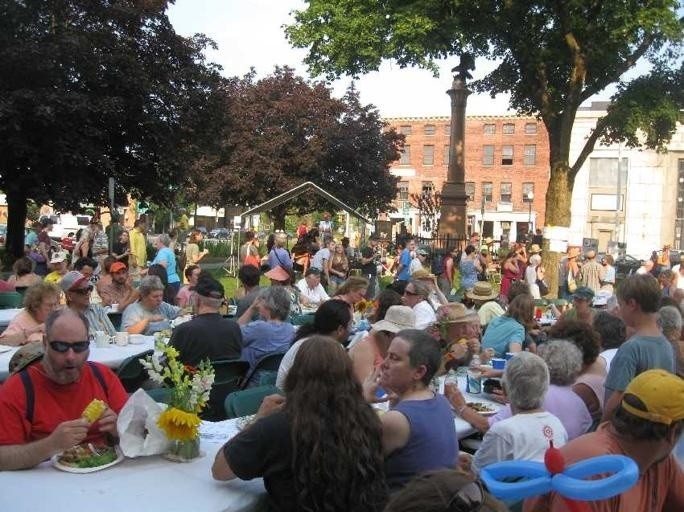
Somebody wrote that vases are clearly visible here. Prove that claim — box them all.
[164,437,200,461]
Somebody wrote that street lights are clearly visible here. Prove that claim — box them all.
[527,191,534,233]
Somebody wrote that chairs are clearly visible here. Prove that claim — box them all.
[241,352,285,390]
[211,359,251,418]
[147,387,177,404]
[117,350,154,393]
[224,385,279,418]
[0,291,23,308]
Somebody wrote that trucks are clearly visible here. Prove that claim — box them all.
[39,215,92,241]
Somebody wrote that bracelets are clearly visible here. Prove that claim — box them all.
[458,404,468,417]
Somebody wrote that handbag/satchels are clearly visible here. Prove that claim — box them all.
[244,245,259,271]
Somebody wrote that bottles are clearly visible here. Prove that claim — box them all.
[444,369,458,398]
[466,355,482,394]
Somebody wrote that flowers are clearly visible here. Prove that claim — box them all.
[138,330,216,443]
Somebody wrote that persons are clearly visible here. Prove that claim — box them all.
[328,245,348,288]
[128,218,147,273]
[525,256,548,302]
[105,211,123,239]
[555,287,599,330]
[22,222,38,253]
[558,246,573,301]
[658,244,670,266]
[59,270,115,332]
[481,294,536,359]
[634,261,654,276]
[530,243,547,294]
[76,225,96,260]
[500,252,519,299]
[481,236,493,252]
[62,232,77,250]
[477,247,490,280]
[368,292,402,324]
[593,312,627,373]
[166,278,243,362]
[387,470,504,512]
[412,268,447,315]
[297,221,309,244]
[240,232,260,270]
[277,300,353,389]
[460,352,567,478]
[95,224,107,263]
[238,285,295,360]
[134,214,146,226]
[448,341,593,438]
[233,264,264,318]
[599,255,616,306]
[341,236,354,258]
[0,281,55,346]
[403,280,435,330]
[175,264,202,309]
[308,227,320,254]
[459,247,483,289]
[44,252,68,301]
[430,302,478,375]
[0,306,131,471]
[263,264,301,316]
[151,234,178,303]
[297,269,330,314]
[293,239,309,274]
[673,252,684,290]
[319,213,333,244]
[563,248,582,299]
[656,304,684,374]
[348,305,416,380]
[508,284,538,352]
[515,226,532,243]
[333,276,370,309]
[467,310,495,363]
[396,237,416,294]
[8,259,40,294]
[267,232,294,283]
[311,238,336,287]
[466,281,505,326]
[184,229,208,268]
[177,208,189,230]
[438,244,459,298]
[111,230,133,270]
[674,289,684,314]
[600,274,674,415]
[374,328,459,481]
[98,260,135,309]
[533,227,544,243]
[118,275,188,333]
[470,231,480,248]
[252,237,261,249]
[513,243,526,281]
[412,249,427,271]
[360,232,381,296]
[660,269,676,297]
[210,334,385,511]
[549,319,611,423]
[496,239,511,256]
[99,255,118,283]
[578,252,603,296]
[72,257,100,306]
[522,369,684,512]
[650,249,663,279]
[36,218,55,259]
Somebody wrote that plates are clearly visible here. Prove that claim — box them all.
[50,449,126,474]
[475,403,501,416]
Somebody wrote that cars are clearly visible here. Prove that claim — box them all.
[208,229,229,239]
[613,253,644,274]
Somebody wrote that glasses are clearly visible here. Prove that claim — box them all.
[443,478,486,512]
[50,340,91,353]
[70,285,94,295]
[404,290,420,296]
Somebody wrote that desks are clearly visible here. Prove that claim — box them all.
[0,325,171,380]
[0,365,509,511]
[1,302,132,327]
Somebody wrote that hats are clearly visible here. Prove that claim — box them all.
[418,249,428,256]
[465,281,497,300]
[60,270,87,292]
[432,301,478,326]
[306,267,321,275]
[8,342,45,375]
[188,278,226,299]
[620,367,683,426]
[586,250,595,259]
[263,265,289,282]
[50,251,67,263]
[565,248,581,259]
[571,287,596,301]
[108,261,127,273]
[371,304,419,333]
[528,243,544,254]
[485,236,493,244]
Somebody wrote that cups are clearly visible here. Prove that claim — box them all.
[492,358,505,369]
[504,352,515,359]
[115,331,129,346]
